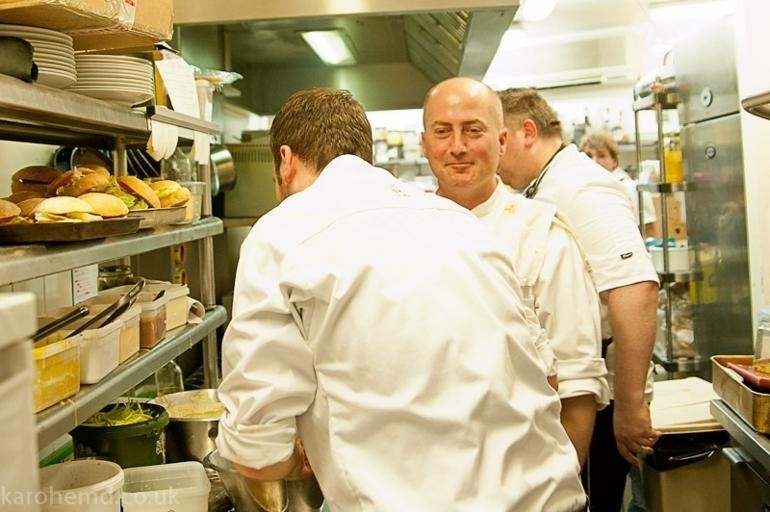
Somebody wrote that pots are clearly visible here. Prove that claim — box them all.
[209,144,237,198]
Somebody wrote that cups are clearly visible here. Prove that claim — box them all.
[161,158,192,188]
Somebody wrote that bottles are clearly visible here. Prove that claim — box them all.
[153,362,184,394]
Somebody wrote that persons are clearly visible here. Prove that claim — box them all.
[578,132,657,242]
[215,88,588,512]
[490,86,661,512]
[425,76,609,475]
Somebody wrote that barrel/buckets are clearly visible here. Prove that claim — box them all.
[68,401,169,470]
[41,459,126,511]
[150,388,226,485]
[121,461,210,512]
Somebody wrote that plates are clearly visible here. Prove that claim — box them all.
[1,24,155,107]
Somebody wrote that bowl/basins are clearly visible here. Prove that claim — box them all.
[202,452,321,512]
[53,144,161,178]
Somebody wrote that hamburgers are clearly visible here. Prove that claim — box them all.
[0,163,190,226]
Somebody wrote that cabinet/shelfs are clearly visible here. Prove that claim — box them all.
[1,72,227,454]
